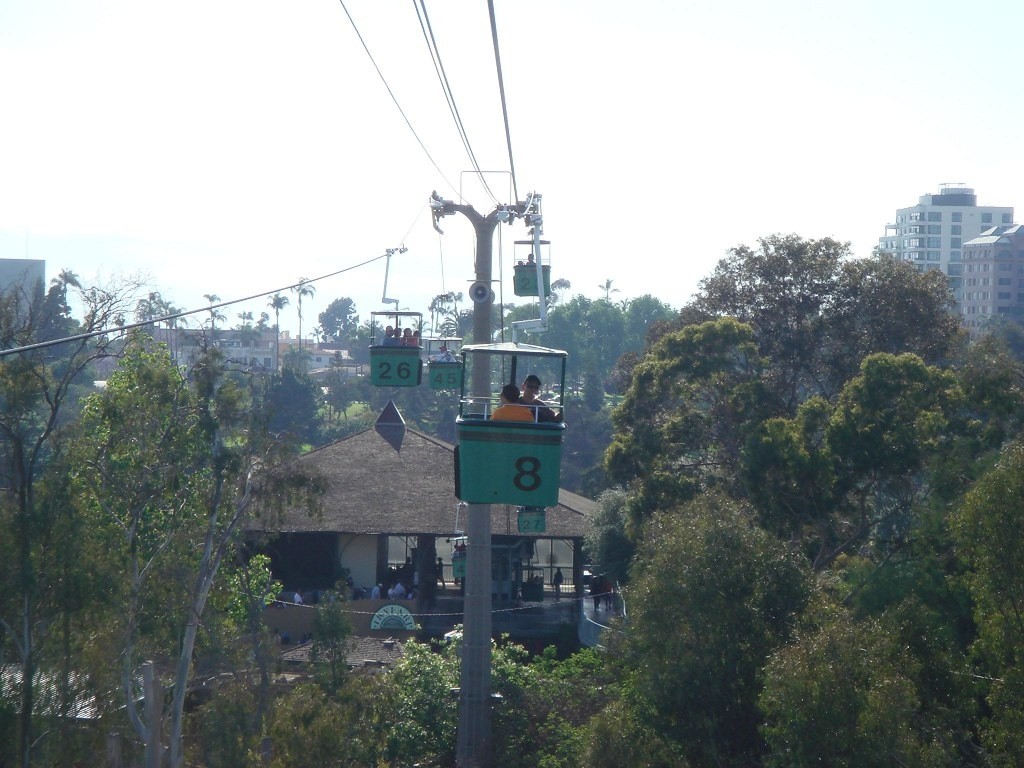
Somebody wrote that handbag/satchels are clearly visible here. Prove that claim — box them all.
[607,594,614,602]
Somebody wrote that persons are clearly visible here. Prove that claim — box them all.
[262,545,462,647]
[381,325,457,362]
[490,385,533,422]
[554,566,615,612]
[517,375,563,423]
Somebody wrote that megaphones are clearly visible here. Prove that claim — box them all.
[469,281,495,304]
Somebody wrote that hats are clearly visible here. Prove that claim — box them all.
[416,330,421,336]
[439,346,447,351]
[503,384,520,400]
[526,375,540,382]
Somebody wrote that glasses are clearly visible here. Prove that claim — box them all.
[527,385,539,389]
